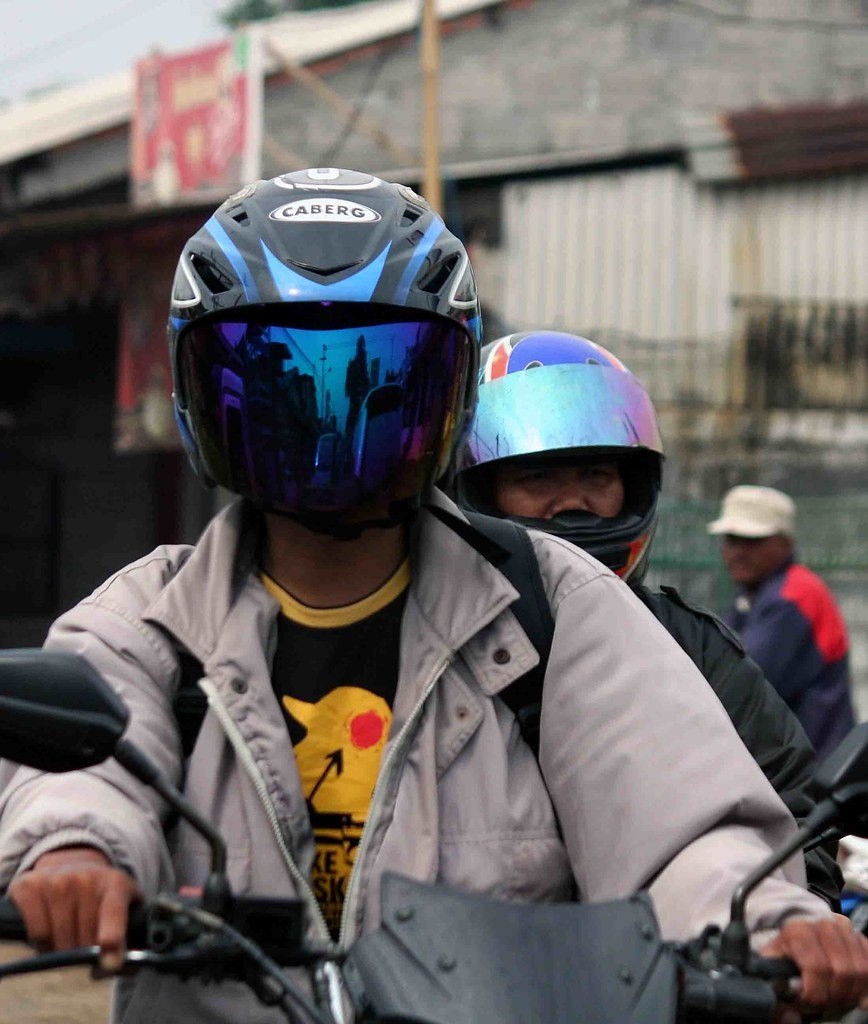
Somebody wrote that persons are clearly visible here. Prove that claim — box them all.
[440,328,845,912]
[0,165,868,1024]
[704,483,856,764]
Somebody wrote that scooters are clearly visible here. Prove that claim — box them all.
[0,651,868,1024]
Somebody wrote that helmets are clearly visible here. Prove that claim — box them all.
[454,330,667,589]
[166,167,484,507]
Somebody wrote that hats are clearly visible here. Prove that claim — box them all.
[706,484,797,539]
